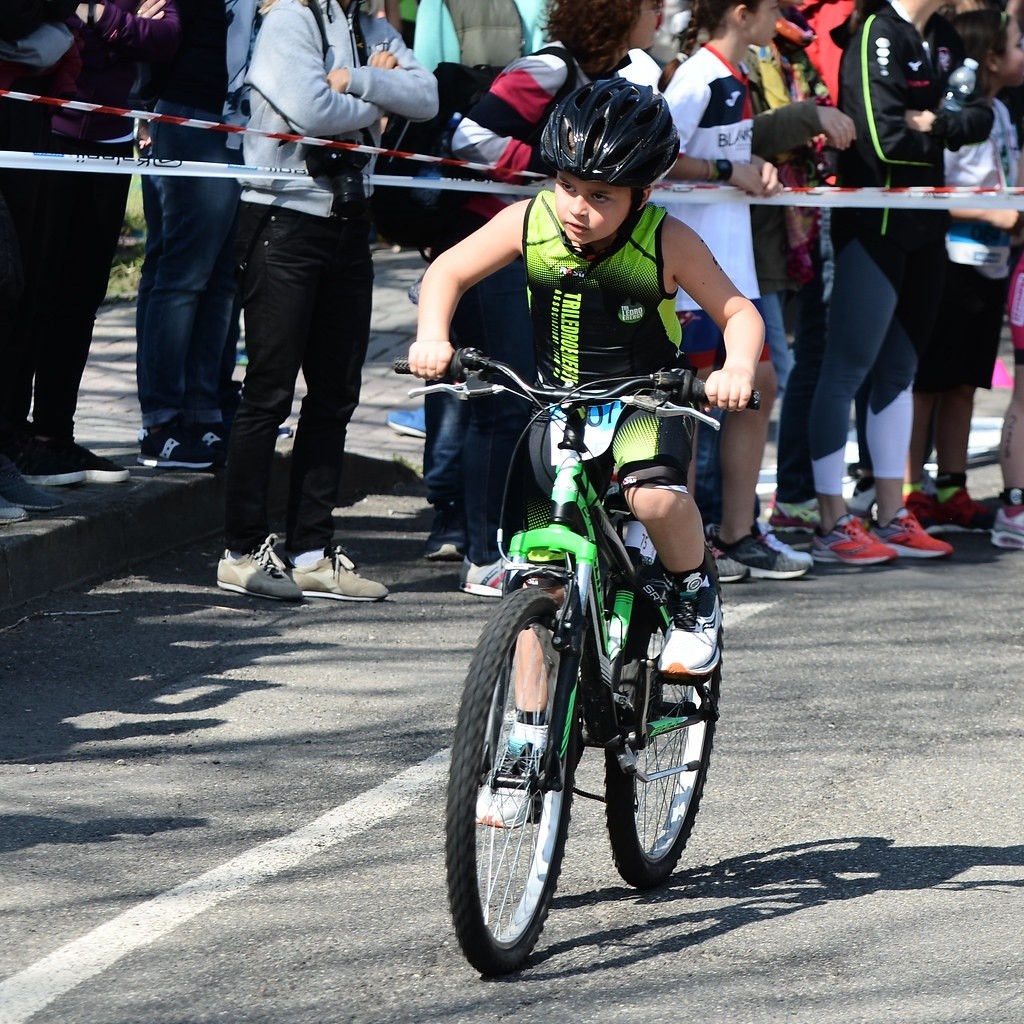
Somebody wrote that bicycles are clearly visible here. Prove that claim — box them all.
[391,346,760,979]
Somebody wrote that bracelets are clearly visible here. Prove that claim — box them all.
[706,159,713,180]
[710,161,719,182]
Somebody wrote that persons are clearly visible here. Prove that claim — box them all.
[408,79,766,831]
[0,0,1023,602]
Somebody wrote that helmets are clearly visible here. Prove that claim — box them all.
[541,77,681,187]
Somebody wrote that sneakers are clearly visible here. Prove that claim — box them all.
[1,425,86,486]
[707,521,813,579]
[218,532,302,601]
[813,514,897,565]
[901,482,961,533]
[389,408,428,440]
[1,498,29,524]
[991,508,1024,549]
[137,427,213,469]
[292,546,388,602]
[189,425,228,461]
[1,455,64,509]
[460,555,506,597]
[423,537,465,560]
[845,462,872,481]
[939,479,994,534]
[477,721,551,827]
[706,541,751,584]
[657,588,723,677]
[870,508,954,558]
[43,436,130,482]
[847,477,878,515]
[770,493,869,535]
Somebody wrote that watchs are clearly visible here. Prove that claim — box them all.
[717,160,732,181]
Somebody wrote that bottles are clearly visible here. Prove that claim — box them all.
[937,58,979,113]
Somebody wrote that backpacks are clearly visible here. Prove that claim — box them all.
[376,47,577,262]
